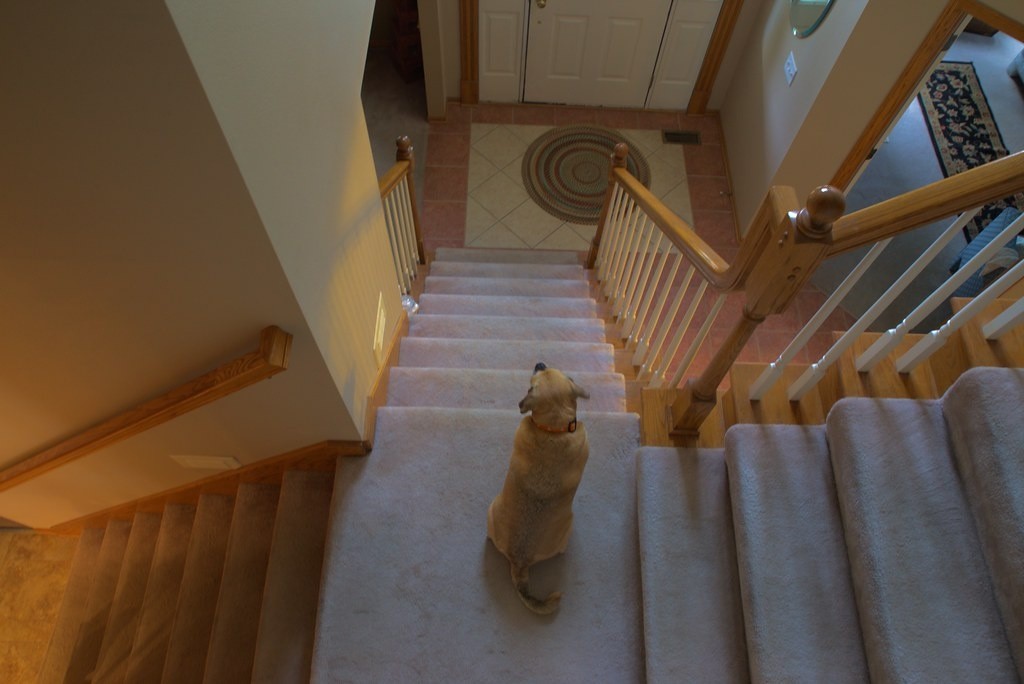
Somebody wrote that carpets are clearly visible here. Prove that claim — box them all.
[521,125,654,224]
[915,59,1024,243]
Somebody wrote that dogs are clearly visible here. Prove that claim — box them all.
[482,362,591,616]
